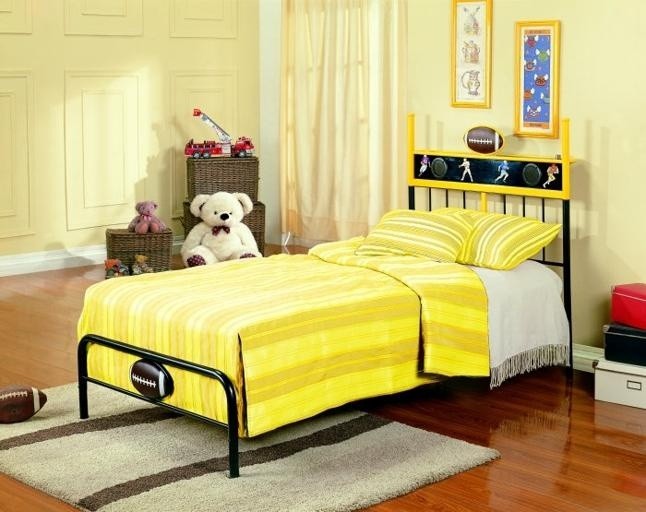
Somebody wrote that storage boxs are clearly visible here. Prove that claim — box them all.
[593,282,646,410]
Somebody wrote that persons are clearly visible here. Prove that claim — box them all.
[418,155,431,177]
[495,160,509,183]
[458,158,473,182]
[543,163,559,188]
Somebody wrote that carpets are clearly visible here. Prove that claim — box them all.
[0,379,500,512]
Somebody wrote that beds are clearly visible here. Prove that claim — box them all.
[78,115,570,479]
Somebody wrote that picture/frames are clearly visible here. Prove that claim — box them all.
[514,20,561,139]
[452,0,493,107]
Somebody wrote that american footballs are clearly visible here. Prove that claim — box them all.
[129,361,169,399]
[2,386,48,422]
[462,126,504,154]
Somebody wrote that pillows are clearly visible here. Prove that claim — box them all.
[432,208,562,270]
[356,210,473,263]
